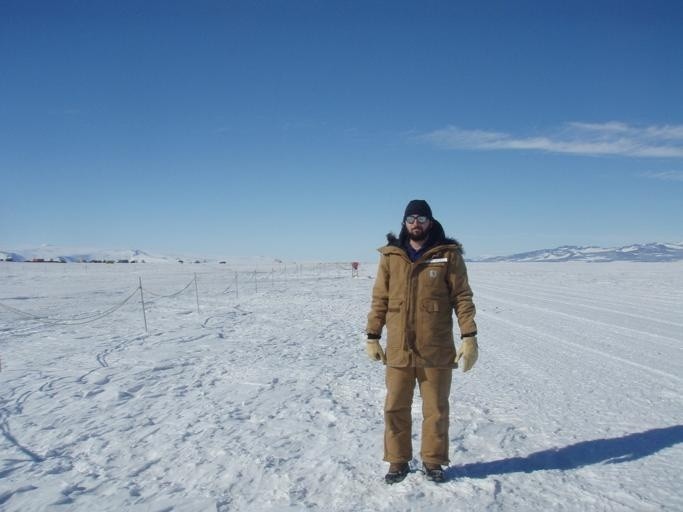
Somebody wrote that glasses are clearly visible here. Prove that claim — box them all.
[404,215,427,224]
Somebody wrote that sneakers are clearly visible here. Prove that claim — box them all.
[421,462,446,483]
[384,462,411,484]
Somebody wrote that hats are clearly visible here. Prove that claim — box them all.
[404,199,432,219]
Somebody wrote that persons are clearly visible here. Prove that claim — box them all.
[363,198,480,486]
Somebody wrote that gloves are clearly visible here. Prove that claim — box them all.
[454,334,481,372]
[363,339,385,364]
